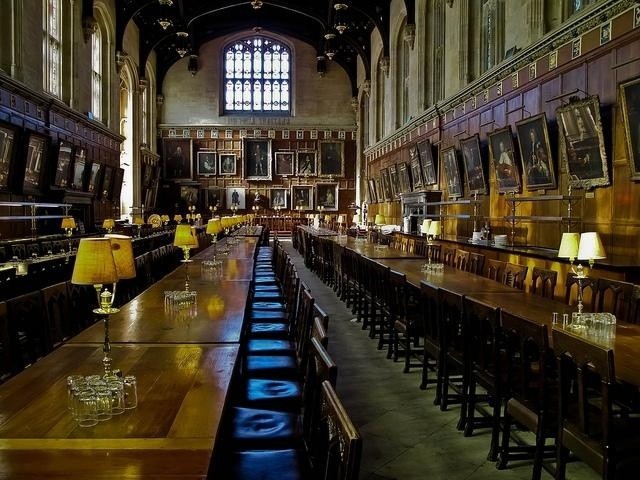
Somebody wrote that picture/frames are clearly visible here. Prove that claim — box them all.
[367,74,639,206]
[141,129,357,212]
[1,121,125,204]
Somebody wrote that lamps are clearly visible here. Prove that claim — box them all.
[156,0,175,30]
[307,214,361,242]
[374,214,388,249]
[324,28,337,61]
[421,219,444,270]
[187,39,201,77]
[174,27,191,58]
[334,0,349,34]
[316,51,326,79]
[53,212,257,368]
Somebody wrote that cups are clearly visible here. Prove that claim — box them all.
[421,263,445,276]
[164,289,197,311]
[335,234,392,252]
[569,311,616,341]
[62,374,138,428]
[165,309,196,329]
[12,229,169,265]
[426,273,445,284]
[202,270,224,281]
[201,234,245,270]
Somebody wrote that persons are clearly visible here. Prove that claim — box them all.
[231,189,240,206]
[273,191,285,207]
[526,128,549,185]
[300,156,312,174]
[169,146,185,178]
[298,190,305,206]
[497,141,512,181]
[223,157,234,172]
[204,156,214,173]
[324,189,335,206]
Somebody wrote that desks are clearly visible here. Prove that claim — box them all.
[0,341,244,479]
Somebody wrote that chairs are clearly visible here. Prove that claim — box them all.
[552,326,640,479]
[496,308,568,480]
[216,336,338,450]
[291,224,391,350]
[386,270,520,463]
[207,381,361,480]
[238,231,330,413]
[391,225,639,389]
